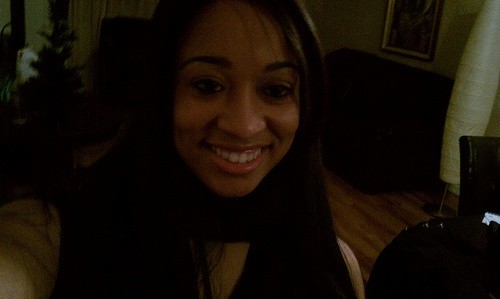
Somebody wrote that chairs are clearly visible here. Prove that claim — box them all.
[457,135,500,219]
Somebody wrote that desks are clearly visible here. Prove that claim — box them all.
[0,95,120,204]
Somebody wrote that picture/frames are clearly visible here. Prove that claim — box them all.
[381,0,444,62]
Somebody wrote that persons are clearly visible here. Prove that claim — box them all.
[1,0,370,299]
